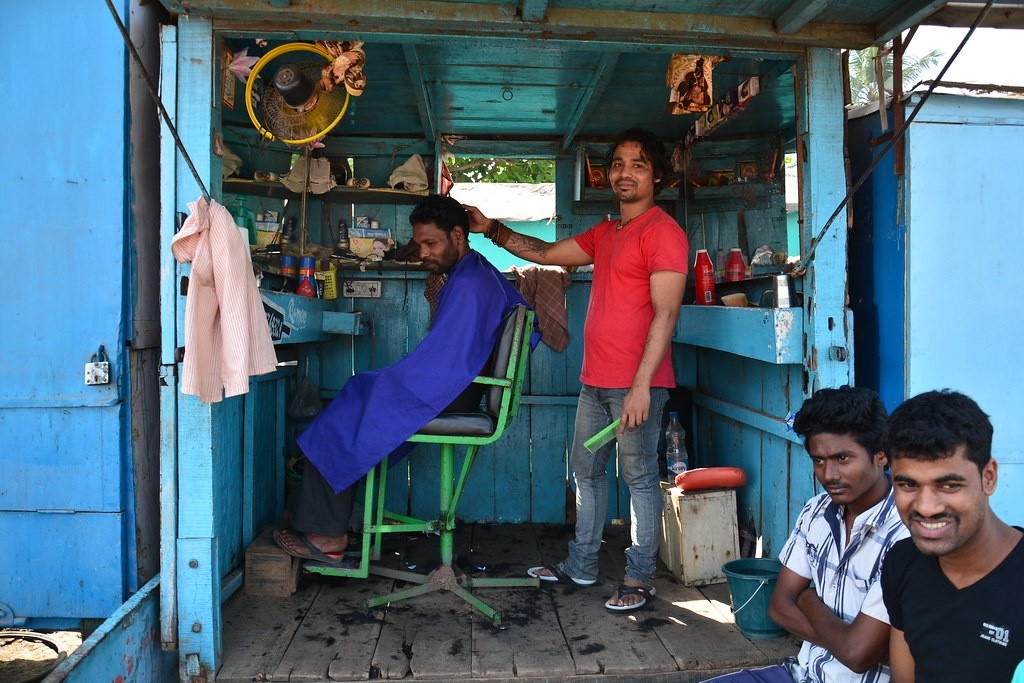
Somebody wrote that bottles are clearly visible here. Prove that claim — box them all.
[725,247,746,281]
[337,223,349,250]
[694,249,717,306]
[666,411,688,484]
[230,195,250,227]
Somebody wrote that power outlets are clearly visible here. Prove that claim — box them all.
[343,281,381,298]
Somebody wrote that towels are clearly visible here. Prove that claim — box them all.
[222,143,242,178]
[280,155,307,193]
[511,262,574,353]
[309,158,335,195]
[388,153,429,192]
[424,272,447,322]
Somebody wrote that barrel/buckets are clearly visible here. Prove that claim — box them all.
[720,557,786,640]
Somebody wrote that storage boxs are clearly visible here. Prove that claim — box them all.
[659,480,739,587]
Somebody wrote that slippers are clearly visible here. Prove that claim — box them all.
[273,528,349,563]
[605,583,656,613]
[527,561,597,585]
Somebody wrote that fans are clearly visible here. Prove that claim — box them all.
[245,44,348,256]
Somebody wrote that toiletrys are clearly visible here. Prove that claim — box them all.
[694,249,716,305]
[726,247,746,281]
[234,195,248,229]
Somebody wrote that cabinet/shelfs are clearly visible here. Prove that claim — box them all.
[221,177,430,270]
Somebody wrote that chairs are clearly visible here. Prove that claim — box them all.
[304,303,541,631]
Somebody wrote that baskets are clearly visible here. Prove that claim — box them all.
[314,261,337,299]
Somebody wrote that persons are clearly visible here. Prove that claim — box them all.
[695,384,911,683]
[881,388,1024,683]
[271,196,508,568]
[461,126,688,610]
